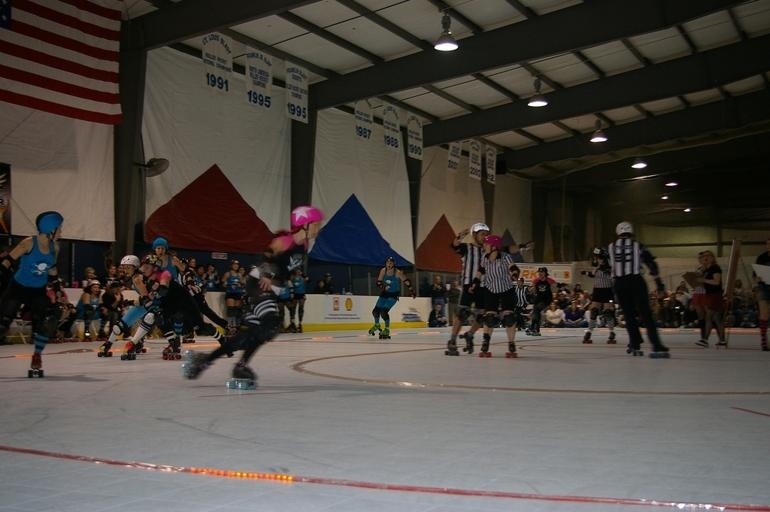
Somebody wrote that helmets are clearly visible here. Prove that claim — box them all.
[37,211,63,233]
[484,235,501,247]
[231,257,240,264]
[537,268,547,272]
[471,223,490,234]
[616,222,634,235]
[385,256,394,261]
[87,238,188,310]
[290,205,323,233]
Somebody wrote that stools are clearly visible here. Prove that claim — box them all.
[71,319,98,340]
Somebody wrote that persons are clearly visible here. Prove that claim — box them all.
[694,251,727,348]
[30,237,310,360]
[369,257,416,340]
[468,234,520,357]
[183,208,324,390]
[324,273,335,295]
[719,280,758,328]
[649,280,698,329]
[444,223,491,356]
[581,247,616,344]
[691,251,720,342]
[429,277,460,327]
[0,211,68,377]
[512,283,625,328]
[609,222,671,359]
[525,267,556,336]
[314,280,330,295]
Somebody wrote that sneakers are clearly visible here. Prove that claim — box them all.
[695,340,727,348]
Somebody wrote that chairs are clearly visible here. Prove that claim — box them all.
[5,312,33,344]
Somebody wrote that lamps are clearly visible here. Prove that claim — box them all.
[589,119,608,143]
[527,78,549,108]
[658,176,692,213]
[433,15,459,52]
[630,157,647,169]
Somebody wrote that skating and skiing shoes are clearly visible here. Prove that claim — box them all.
[369,324,382,335]
[28,324,258,390]
[380,328,391,338]
[445,326,670,358]
